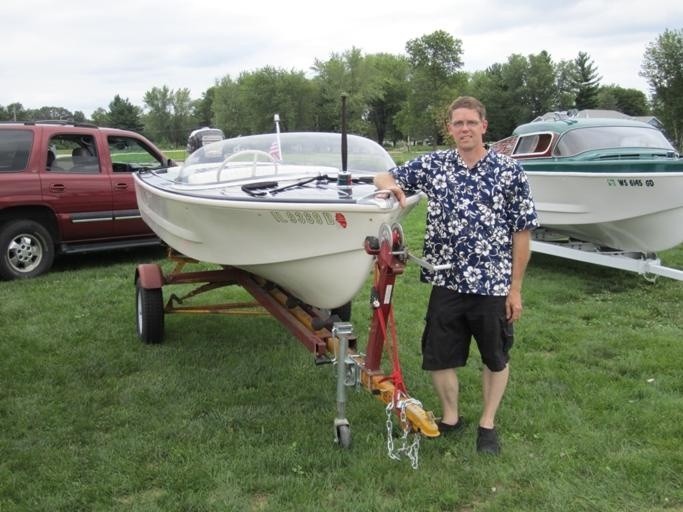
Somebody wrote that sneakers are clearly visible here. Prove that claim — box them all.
[475,424,500,460]
[428,415,465,439]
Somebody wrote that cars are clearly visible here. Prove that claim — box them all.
[0,120,179,279]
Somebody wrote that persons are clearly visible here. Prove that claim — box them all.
[185,118,226,162]
[372,97,542,454]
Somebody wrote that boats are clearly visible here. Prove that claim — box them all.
[132,132,423,310]
[506,109,683,254]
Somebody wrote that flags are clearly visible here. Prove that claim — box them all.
[267,135,280,164]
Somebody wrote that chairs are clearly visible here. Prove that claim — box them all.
[70,149,101,172]
[46,150,66,171]
